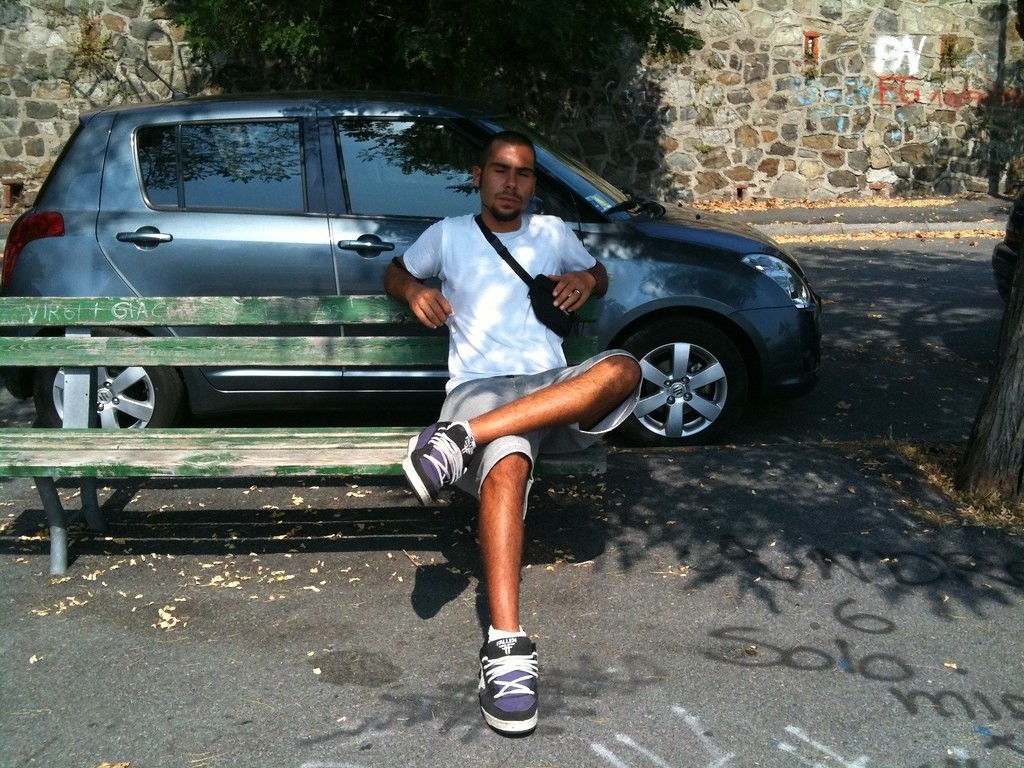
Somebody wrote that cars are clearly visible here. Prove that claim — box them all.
[0,87,823,454]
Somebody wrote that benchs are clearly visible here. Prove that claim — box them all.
[0,294,607,575]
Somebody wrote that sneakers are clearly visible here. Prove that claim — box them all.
[401,421,477,506]
[477,636,538,733]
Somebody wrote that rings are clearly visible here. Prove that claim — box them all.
[574,289,581,296]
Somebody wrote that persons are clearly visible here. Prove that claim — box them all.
[384,130,644,735]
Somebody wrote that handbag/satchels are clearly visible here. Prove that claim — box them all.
[529,274,576,337]
